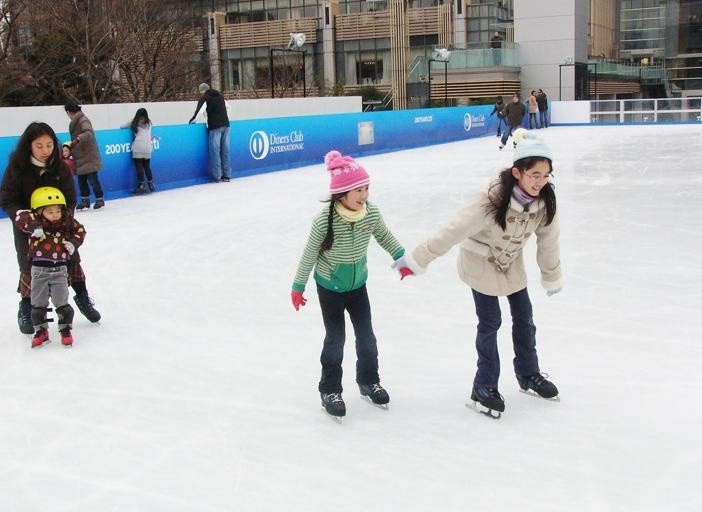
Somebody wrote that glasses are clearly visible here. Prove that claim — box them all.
[519,169,555,183]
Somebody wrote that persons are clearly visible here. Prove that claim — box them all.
[64,101,105,212]
[490,95,513,137]
[15,186,87,347]
[291,150,416,424]
[189,83,232,184]
[498,93,527,149]
[391,125,565,419]
[491,31,503,67]
[0,120,101,336]
[62,140,77,208]
[536,88,549,128]
[120,108,156,194]
[524,90,540,129]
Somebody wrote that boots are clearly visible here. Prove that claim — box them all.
[149,181,155,192]
[73,296,102,326]
[134,182,146,196]
[17,301,35,335]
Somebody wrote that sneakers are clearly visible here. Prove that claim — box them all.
[465,383,505,419]
[92,198,105,212]
[357,381,390,410]
[320,390,347,423]
[208,177,220,184]
[60,330,74,347]
[515,370,560,401]
[76,198,91,212]
[31,328,50,349]
[513,141,517,148]
[499,144,506,150]
[221,176,231,182]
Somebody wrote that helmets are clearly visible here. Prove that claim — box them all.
[30,186,67,211]
[62,141,73,148]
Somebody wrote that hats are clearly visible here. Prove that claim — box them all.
[510,127,554,164]
[513,93,520,97]
[198,83,211,92]
[325,150,370,195]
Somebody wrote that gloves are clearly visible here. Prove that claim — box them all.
[64,241,76,256]
[291,292,307,311]
[70,138,79,146]
[391,256,414,280]
[31,228,44,238]
[546,289,562,296]
